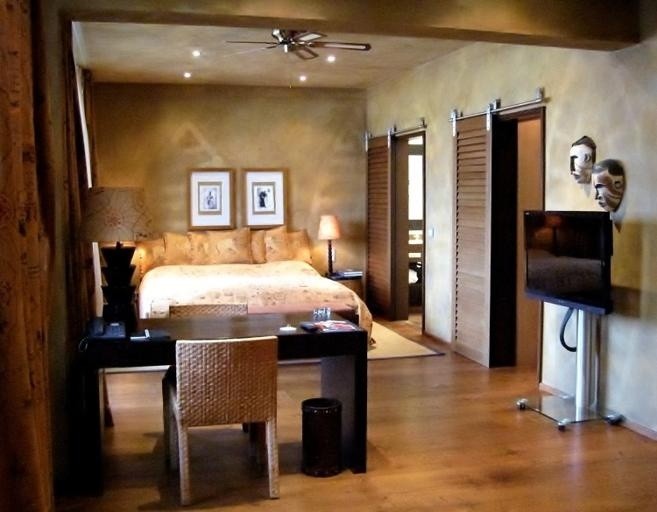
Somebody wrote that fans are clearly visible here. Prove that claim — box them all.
[223,28,371,60]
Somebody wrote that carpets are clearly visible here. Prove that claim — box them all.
[99,320,445,374]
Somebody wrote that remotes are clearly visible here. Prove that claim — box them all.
[298,320,320,333]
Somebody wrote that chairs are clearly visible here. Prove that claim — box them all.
[169,305,254,434]
[161,334,278,506]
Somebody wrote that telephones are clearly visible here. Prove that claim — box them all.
[78,318,124,354]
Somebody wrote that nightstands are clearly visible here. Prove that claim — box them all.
[339,278,364,299]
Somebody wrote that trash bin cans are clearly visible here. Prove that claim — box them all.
[302,397,344,477]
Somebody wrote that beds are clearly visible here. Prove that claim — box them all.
[139,262,375,351]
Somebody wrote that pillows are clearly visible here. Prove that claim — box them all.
[162,230,191,265]
[251,230,265,264]
[289,228,312,261]
[188,232,207,265]
[265,226,292,261]
[206,229,251,263]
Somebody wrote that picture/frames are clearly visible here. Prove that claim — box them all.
[186,167,237,231]
[240,166,291,228]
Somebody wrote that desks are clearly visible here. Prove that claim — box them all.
[78,313,367,474]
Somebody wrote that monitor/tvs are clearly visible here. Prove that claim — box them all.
[523,209,616,316]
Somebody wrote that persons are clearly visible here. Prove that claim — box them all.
[592,159,625,214]
[570,137,595,186]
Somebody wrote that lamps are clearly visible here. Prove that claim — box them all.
[318,214,345,280]
[79,188,159,334]
